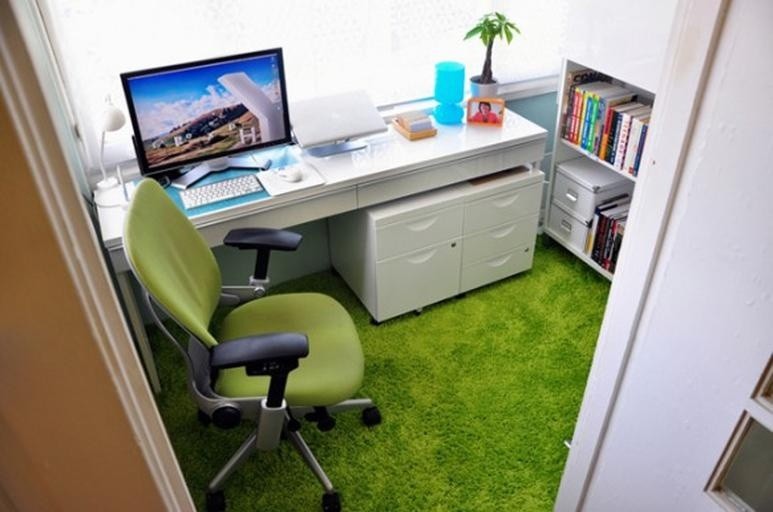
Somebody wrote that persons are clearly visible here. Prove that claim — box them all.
[471,102,496,123]
[562,70,652,177]
[585,193,632,274]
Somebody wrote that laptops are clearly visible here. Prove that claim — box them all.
[288,86,388,149]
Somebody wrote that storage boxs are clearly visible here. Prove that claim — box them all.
[547,156,632,254]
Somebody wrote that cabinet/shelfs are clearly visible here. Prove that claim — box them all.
[325,163,546,328]
[543,56,657,285]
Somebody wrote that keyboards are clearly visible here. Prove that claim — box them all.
[179,174,264,210]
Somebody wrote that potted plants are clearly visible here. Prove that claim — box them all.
[460,9,520,103]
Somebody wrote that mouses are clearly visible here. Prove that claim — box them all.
[275,167,302,182]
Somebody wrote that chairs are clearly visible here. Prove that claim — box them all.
[119,175,383,511]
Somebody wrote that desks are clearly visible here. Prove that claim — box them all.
[88,101,551,397]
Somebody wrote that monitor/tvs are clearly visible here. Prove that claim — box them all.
[120,48,292,190]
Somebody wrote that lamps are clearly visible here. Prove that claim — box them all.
[89,92,124,192]
